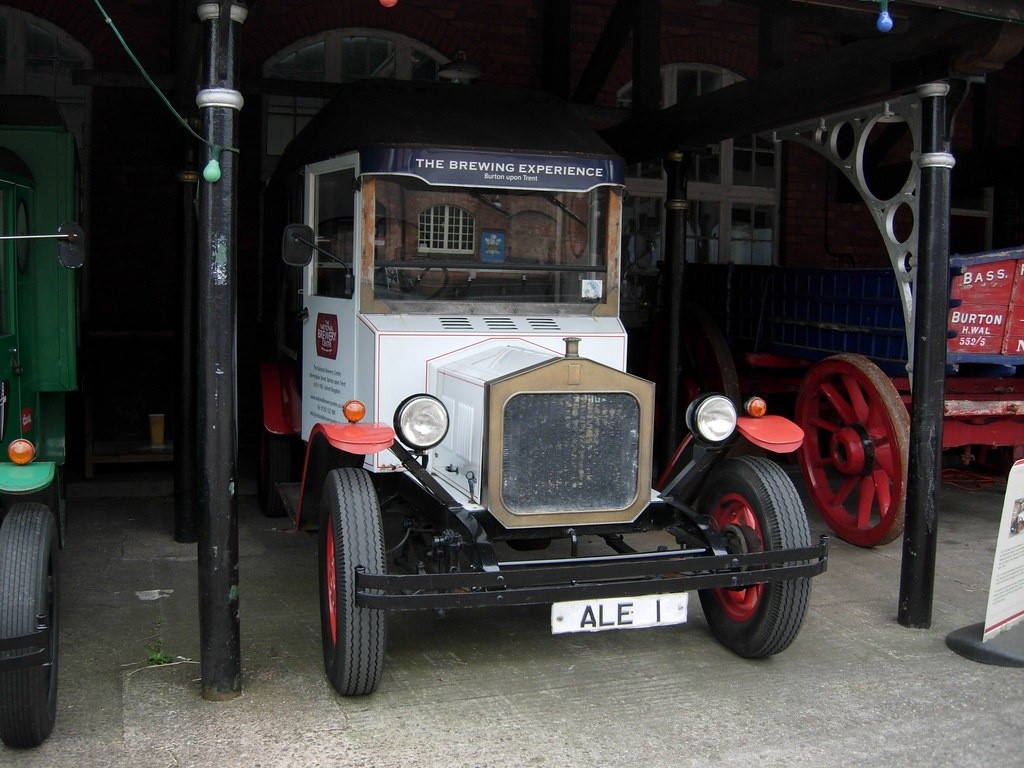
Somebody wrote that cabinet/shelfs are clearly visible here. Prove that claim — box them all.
[79,330,174,479]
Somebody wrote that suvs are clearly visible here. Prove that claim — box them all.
[639,56,1024,552]
[0,115,89,752]
[257,139,831,696]
